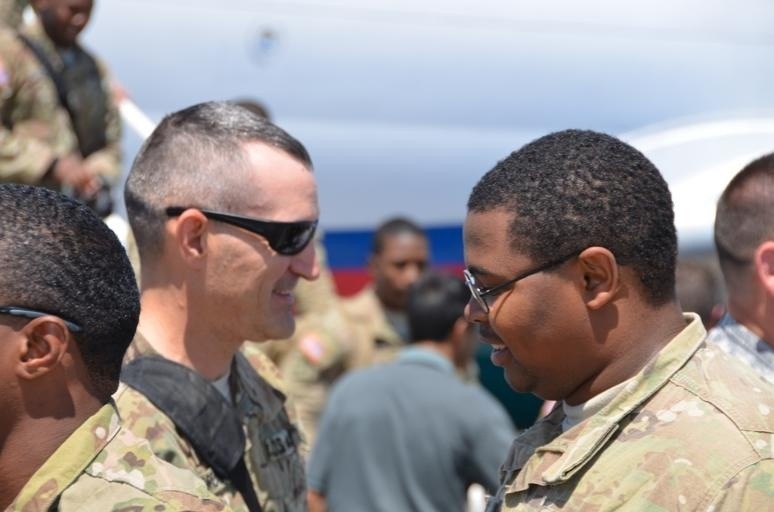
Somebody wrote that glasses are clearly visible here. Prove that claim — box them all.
[167,207,319,256]
[464,257,576,314]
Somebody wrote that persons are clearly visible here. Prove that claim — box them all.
[461,128,774,512]
[303,271,520,512]
[703,151,774,384]
[669,254,729,338]
[1,179,245,511]
[113,96,323,511]
[2,0,122,216]
[283,215,484,444]
